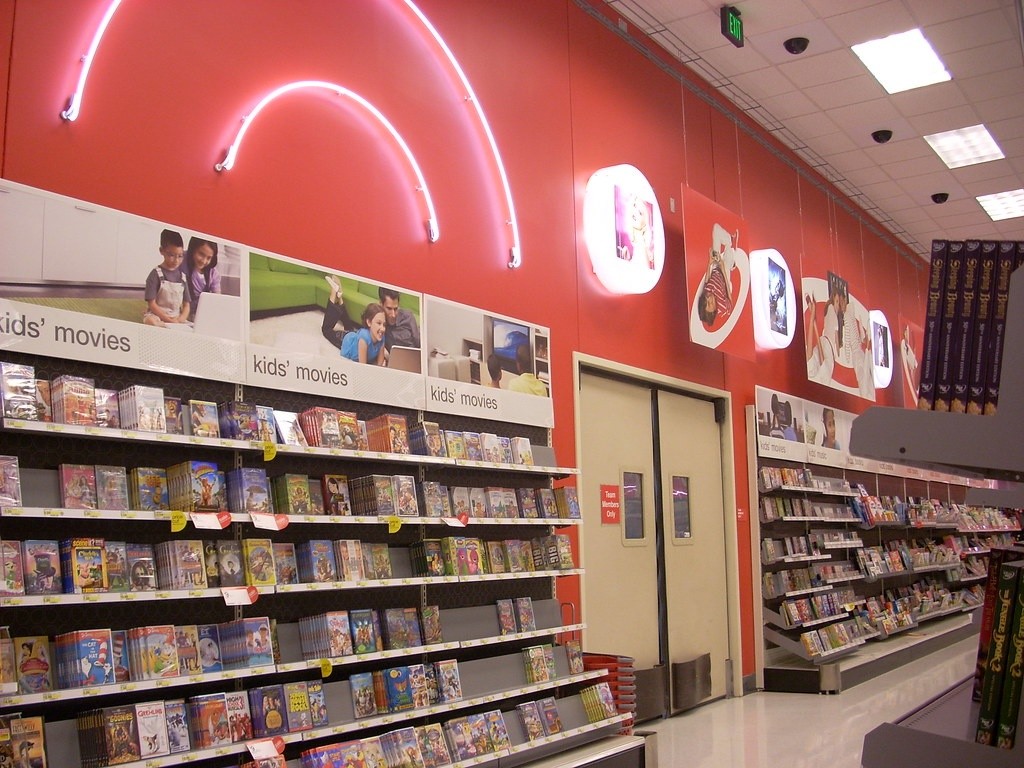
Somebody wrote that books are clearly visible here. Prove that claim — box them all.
[759,466,1021,657]
[1,362,619,768]
[972,541,1024,749]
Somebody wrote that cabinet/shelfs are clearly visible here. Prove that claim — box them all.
[850,264,1024,768]
[746,405,1024,695]
[0,177,658,767]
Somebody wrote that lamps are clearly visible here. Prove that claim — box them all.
[749,248,798,351]
[583,161,665,296]
[870,309,894,391]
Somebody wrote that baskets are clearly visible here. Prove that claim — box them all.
[580,652,637,735]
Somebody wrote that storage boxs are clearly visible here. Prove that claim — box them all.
[0,362,620,767]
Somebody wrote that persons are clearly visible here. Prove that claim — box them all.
[143,227,220,332]
[697,223,741,326]
[771,393,798,442]
[820,408,840,450]
[804,410,817,445]
[618,198,652,269]
[769,280,784,315]
[509,345,548,397]
[322,275,421,366]
[484,354,502,389]
[805,286,919,402]
